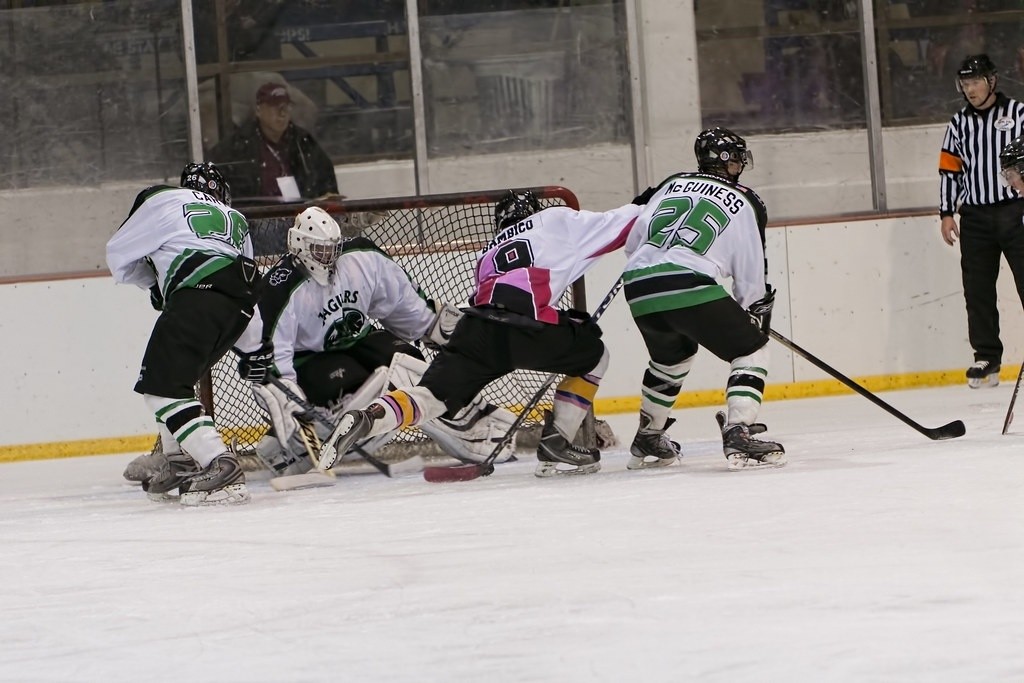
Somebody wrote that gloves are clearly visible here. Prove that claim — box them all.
[148,282,164,311]
[238,341,275,383]
[631,187,657,206]
[746,284,777,336]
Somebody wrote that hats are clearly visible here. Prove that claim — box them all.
[256,84,296,106]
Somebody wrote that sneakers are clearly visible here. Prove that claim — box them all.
[319,403,386,470]
[966,360,1001,388]
[147,446,204,502]
[535,409,601,476]
[179,436,250,507]
[715,411,786,471]
[627,409,683,470]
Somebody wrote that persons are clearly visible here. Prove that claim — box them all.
[624,124,790,471]
[940,52,1024,389]
[317,185,660,478]
[999,135,1024,198]
[205,84,357,254]
[255,207,517,493]
[104,161,264,508]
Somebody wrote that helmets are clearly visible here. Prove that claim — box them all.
[495,189,540,235]
[694,127,747,161]
[180,162,232,208]
[999,135,1024,170]
[287,206,343,287]
[958,54,997,79]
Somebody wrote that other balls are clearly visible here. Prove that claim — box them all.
[481,461,495,477]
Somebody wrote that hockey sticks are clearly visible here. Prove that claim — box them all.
[1002,361,1024,436]
[768,328,966,440]
[230,342,424,478]
[423,275,625,484]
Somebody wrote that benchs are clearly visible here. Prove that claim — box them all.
[0,20,437,192]
